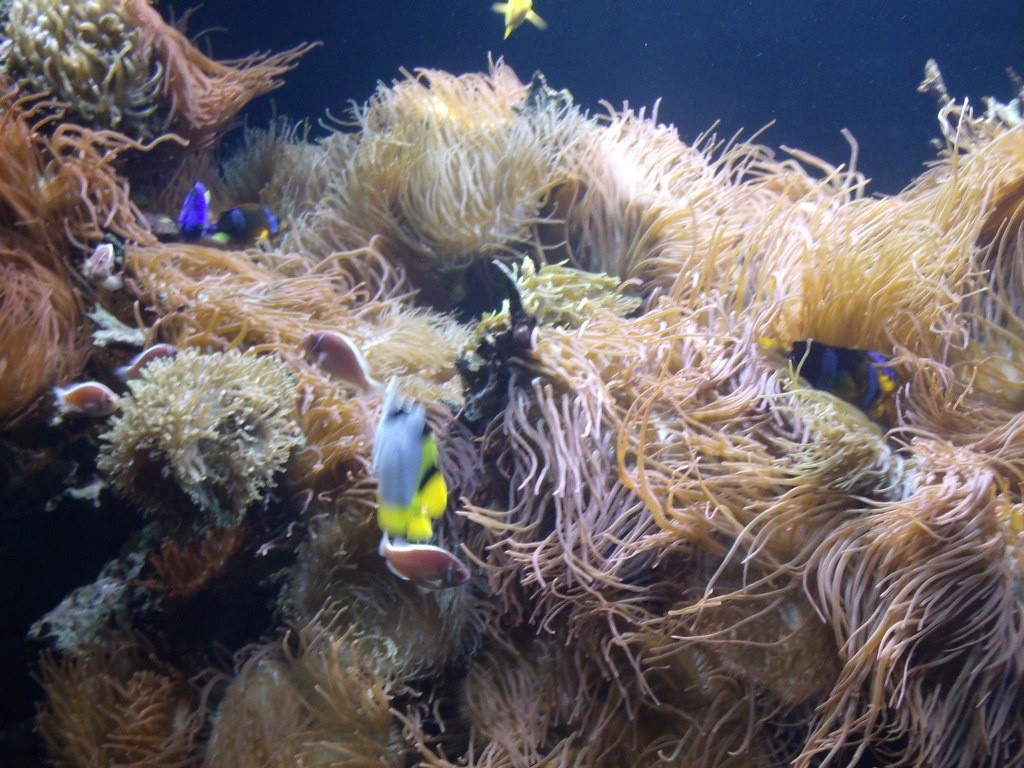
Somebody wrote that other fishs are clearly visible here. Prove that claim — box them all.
[53,0,906,595]
